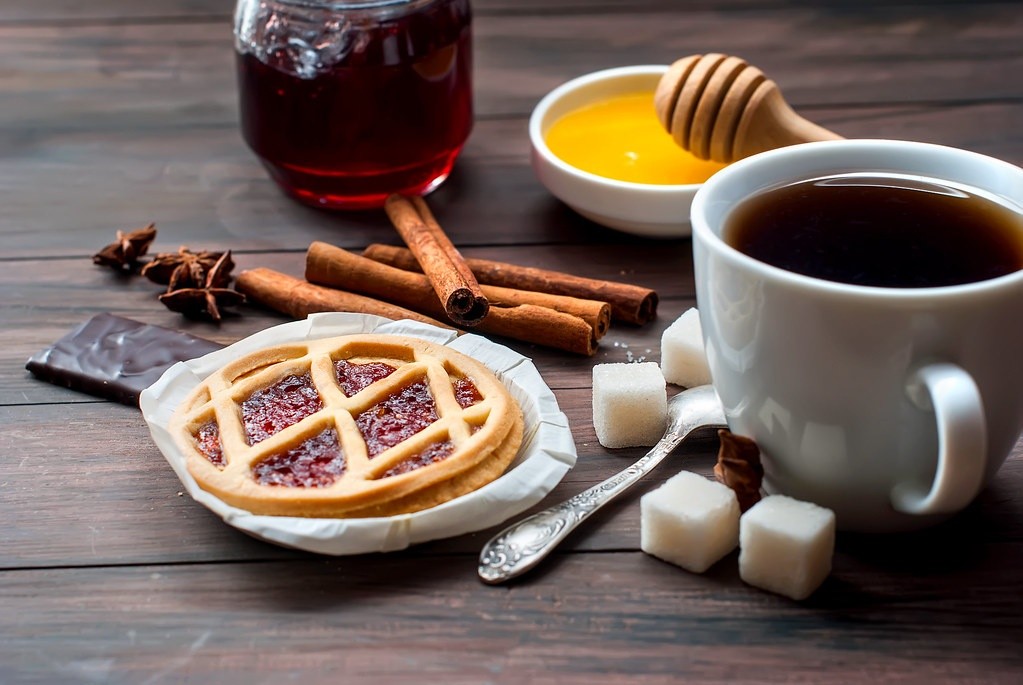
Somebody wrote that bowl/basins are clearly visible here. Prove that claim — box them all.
[527,63,705,238]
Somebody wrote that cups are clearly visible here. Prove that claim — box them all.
[230,0,476,212]
[688,138,1023,538]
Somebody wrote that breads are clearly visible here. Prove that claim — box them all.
[175,331,526,518]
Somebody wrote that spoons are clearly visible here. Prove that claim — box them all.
[478,383,731,585]
